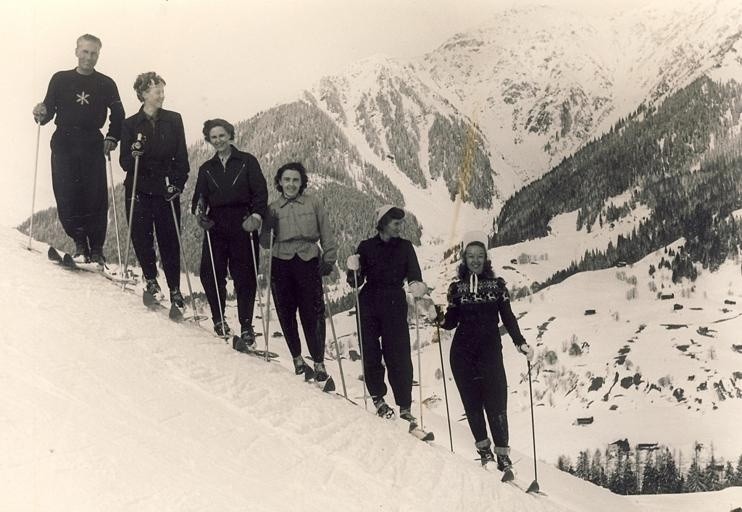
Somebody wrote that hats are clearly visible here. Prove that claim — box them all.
[462,230,489,254]
[373,205,396,227]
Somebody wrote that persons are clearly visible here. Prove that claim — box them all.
[191,118,268,347]
[436,241,530,472]
[34,33,125,264]
[347,204,427,423]
[119,72,190,311]
[259,163,336,381]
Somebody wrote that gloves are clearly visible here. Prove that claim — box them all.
[130,141,144,157]
[346,253,361,271]
[260,230,276,250]
[241,214,263,233]
[316,259,332,276]
[405,281,443,326]
[162,182,180,202]
[197,214,213,229]
[515,343,534,361]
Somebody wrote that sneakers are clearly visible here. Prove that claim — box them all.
[73,241,107,271]
[211,316,230,336]
[146,279,164,302]
[293,355,330,387]
[241,324,255,347]
[371,396,395,421]
[399,405,419,434]
[169,286,187,315]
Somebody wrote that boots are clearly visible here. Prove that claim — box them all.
[494,446,514,473]
[475,439,496,468]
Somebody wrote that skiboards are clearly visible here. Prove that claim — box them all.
[200,312,337,394]
[482,457,538,492]
[47,242,210,325]
[371,406,434,442]
